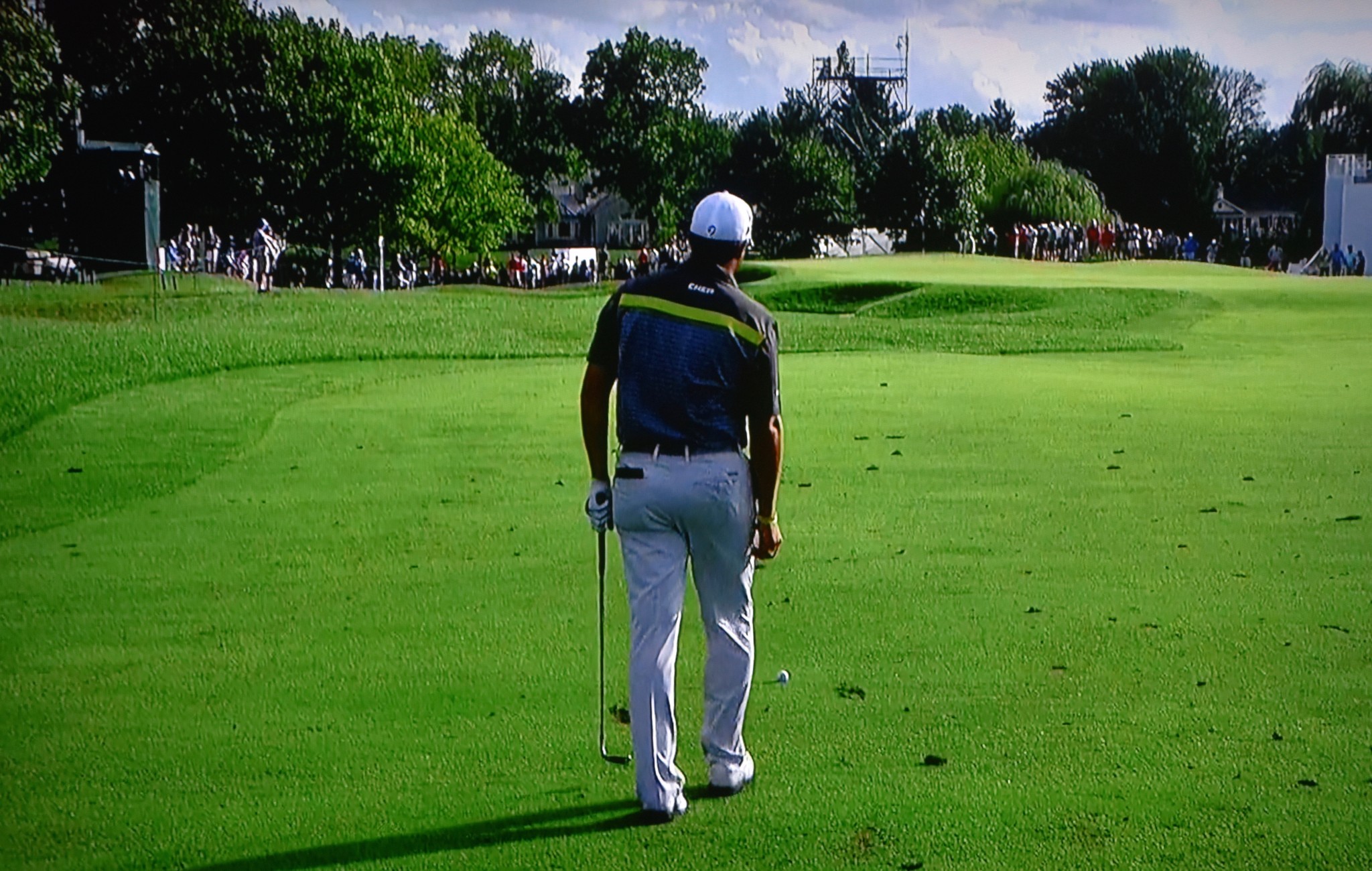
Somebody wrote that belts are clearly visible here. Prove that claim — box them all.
[612,434,743,457]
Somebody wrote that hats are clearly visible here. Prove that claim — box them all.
[685,191,760,252]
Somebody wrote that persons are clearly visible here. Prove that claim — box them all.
[949,213,1366,278]
[577,188,784,824]
[165,218,691,293]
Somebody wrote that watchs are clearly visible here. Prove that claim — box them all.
[754,509,779,527]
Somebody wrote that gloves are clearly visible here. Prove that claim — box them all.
[588,479,616,535]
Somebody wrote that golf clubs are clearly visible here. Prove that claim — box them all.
[595,491,633,764]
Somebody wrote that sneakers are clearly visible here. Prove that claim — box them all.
[639,784,689,820]
[709,748,756,792]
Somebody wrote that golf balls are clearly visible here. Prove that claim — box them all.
[779,670,789,682]
